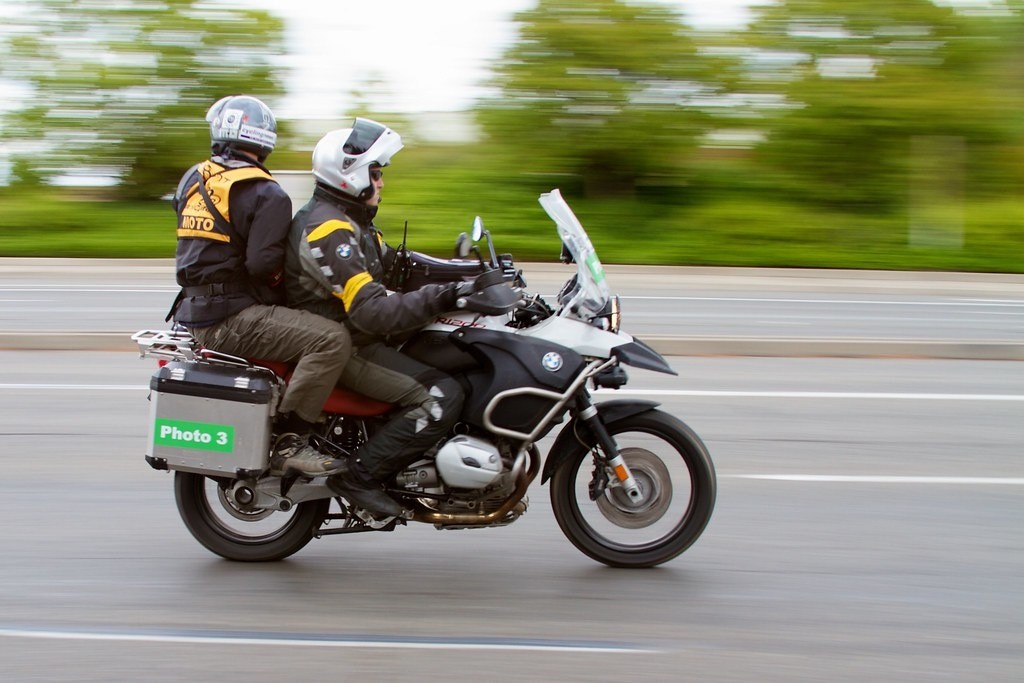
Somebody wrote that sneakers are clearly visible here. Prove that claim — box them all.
[282,446,348,477]
[326,472,401,515]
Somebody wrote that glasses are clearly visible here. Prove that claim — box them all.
[368,170,383,181]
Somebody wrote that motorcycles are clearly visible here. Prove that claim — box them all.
[130,191,717,569]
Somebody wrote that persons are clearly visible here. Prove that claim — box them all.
[171,95,353,479]
[285,115,477,517]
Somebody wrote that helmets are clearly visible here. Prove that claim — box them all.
[206,96,277,153]
[312,117,404,198]
